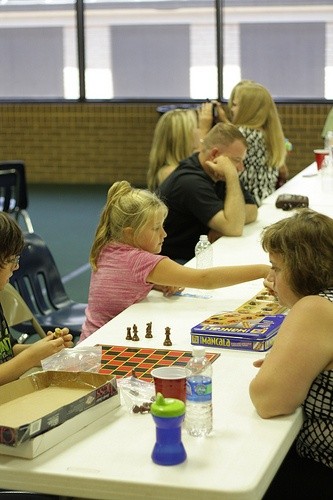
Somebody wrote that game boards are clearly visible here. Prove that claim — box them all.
[95,344,221,384]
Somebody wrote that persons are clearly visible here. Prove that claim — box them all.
[147,80,289,209]
[0,213,74,386]
[248,209,333,500]
[153,123,259,264]
[73,180,273,347]
[321,108,333,147]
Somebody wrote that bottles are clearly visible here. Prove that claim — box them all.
[324,132,333,174]
[150,390,187,465]
[194,234,214,269]
[185,346,214,435]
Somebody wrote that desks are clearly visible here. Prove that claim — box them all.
[0,161,333,500]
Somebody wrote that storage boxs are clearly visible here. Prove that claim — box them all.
[0,369,120,458]
[191,310,286,352]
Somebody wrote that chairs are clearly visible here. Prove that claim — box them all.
[0,161,87,379]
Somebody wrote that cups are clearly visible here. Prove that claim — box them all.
[149,367,192,405]
[313,149,329,169]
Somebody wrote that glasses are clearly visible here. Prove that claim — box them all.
[5,256,20,268]
